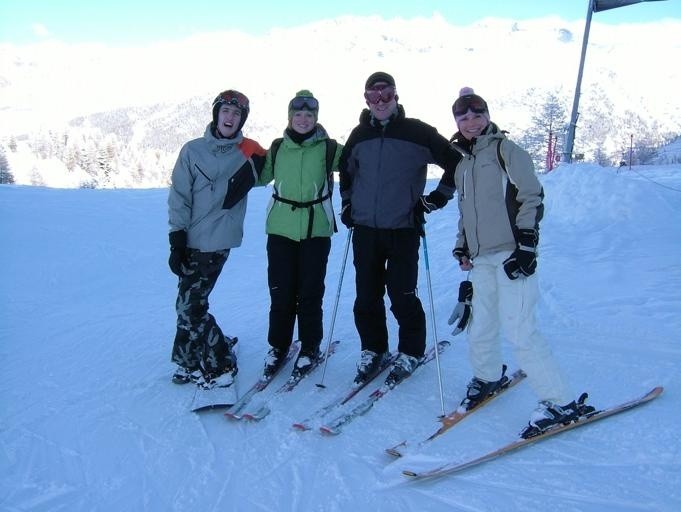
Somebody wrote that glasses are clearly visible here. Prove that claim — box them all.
[363,86,396,105]
[453,97,488,115]
[289,97,319,111]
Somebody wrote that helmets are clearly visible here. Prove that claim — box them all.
[212,89,250,136]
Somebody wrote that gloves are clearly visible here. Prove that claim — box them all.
[448,281,473,336]
[418,190,449,214]
[340,199,354,229]
[515,228,537,277]
[167,230,191,279]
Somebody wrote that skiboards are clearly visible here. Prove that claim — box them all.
[224,337,340,421]
[385,369,664,480]
[292,341,452,435]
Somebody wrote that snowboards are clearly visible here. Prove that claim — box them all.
[189,334,239,415]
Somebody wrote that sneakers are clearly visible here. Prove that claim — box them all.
[293,349,316,375]
[172,365,191,383]
[387,352,422,380]
[467,364,507,402]
[356,350,389,378]
[215,361,237,388]
[522,392,596,437]
[264,346,290,377]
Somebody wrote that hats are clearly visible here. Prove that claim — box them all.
[288,89,319,128]
[365,72,395,89]
[451,87,489,116]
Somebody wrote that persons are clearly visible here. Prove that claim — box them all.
[164,90,268,389]
[254,90,345,375]
[338,71,464,379]
[450,86,577,431]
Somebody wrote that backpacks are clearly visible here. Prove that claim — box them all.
[272,137,339,234]
